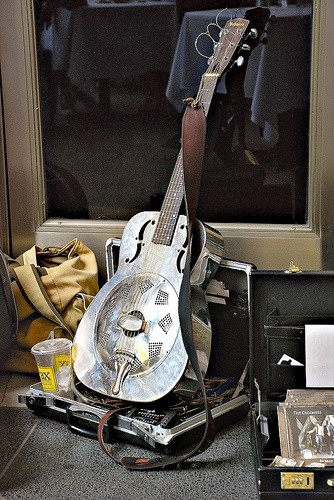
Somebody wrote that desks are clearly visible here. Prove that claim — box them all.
[167,5,312,137]
[47,0,183,132]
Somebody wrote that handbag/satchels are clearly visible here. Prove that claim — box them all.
[3,238,100,375]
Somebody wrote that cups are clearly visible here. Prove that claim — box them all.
[30,336,74,404]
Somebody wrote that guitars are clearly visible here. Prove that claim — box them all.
[215,6,277,129]
[69,6,259,409]
